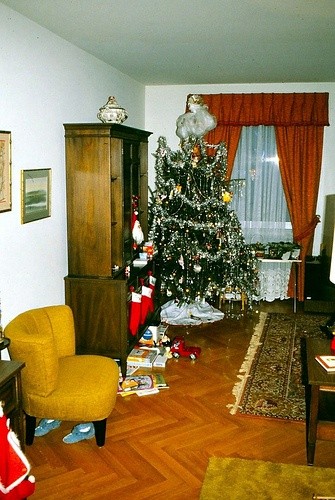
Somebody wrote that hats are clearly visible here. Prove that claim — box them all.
[131,211,145,244]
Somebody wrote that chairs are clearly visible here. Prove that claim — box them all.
[4,305,119,447]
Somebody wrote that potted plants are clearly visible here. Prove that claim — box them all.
[249,241,301,260]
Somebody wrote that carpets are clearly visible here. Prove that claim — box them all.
[226,312,335,422]
[198,456,335,500]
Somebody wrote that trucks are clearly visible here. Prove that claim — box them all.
[169,336,202,360]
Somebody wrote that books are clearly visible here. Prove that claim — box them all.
[117,372,169,398]
[119,366,139,375]
[315,355,335,375]
[126,348,168,368]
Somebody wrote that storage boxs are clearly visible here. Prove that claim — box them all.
[126,325,168,368]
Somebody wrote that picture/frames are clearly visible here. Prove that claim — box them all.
[0,130,12,214]
[21,168,51,224]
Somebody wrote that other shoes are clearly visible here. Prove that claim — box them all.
[34,418,62,437]
[63,423,96,444]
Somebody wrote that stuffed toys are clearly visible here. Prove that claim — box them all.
[131,211,145,250]
[0,401,36,500]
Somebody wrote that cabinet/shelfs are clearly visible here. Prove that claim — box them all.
[0,359,25,454]
[304,256,335,301]
[62,123,154,377]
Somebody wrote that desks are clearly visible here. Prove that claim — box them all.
[219,259,302,313]
[306,337,335,466]
